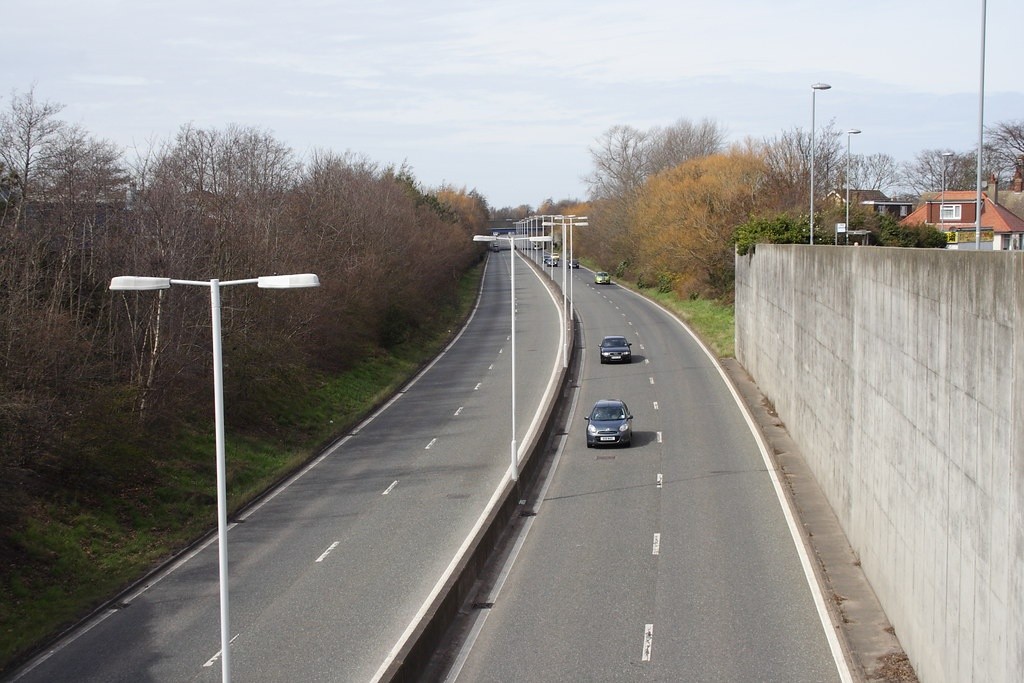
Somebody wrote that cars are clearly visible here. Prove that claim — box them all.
[534,244,541,250]
[543,252,560,267]
[595,272,611,285]
[598,334,633,364]
[569,259,579,269]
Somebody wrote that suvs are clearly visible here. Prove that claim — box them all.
[584,399,633,449]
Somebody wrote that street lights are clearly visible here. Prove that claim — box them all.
[941,153,955,232]
[108,272,321,683]
[845,128,861,245]
[471,234,554,482]
[512,215,589,321]
[808,80,832,245]
